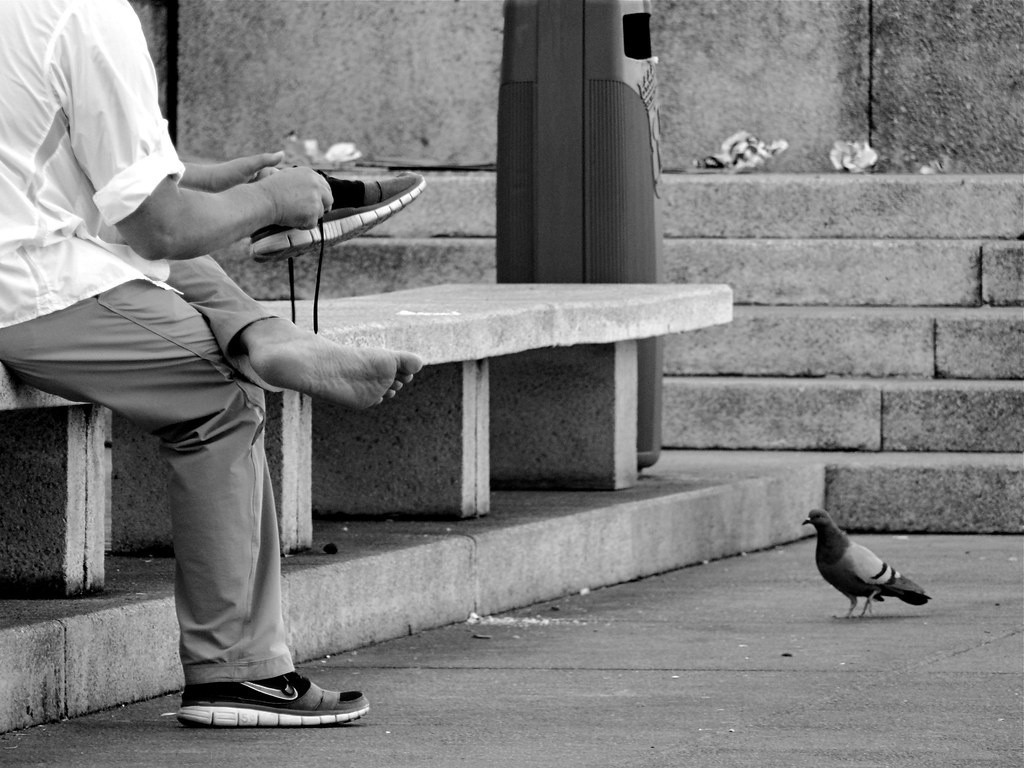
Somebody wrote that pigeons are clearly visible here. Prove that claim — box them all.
[802,508,933,618]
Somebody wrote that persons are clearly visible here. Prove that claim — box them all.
[1,0,422,727]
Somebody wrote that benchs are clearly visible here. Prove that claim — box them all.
[0,282,733,596]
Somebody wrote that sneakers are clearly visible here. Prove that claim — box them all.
[175,669,369,726]
[252,165,428,333]
